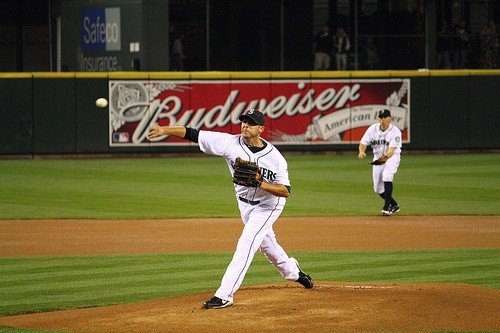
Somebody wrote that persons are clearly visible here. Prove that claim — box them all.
[435,17,477,71]
[146,107,314,309]
[167,22,185,71]
[333,26,350,70]
[312,24,339,71]
[357,109,402,216]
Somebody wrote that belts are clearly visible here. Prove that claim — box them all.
[238,196,261,206]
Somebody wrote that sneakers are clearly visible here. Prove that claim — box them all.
[203,296,234,309]
[290,257,314,289]
[380,206,391,216]
[389,204,400,214]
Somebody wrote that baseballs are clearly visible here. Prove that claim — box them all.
[96,98,108,108]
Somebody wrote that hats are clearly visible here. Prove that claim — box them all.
[239,109,265,125]
[378,109,390,116]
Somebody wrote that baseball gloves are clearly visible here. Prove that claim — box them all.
[231,156,262,190]
[367,155,389,165]
